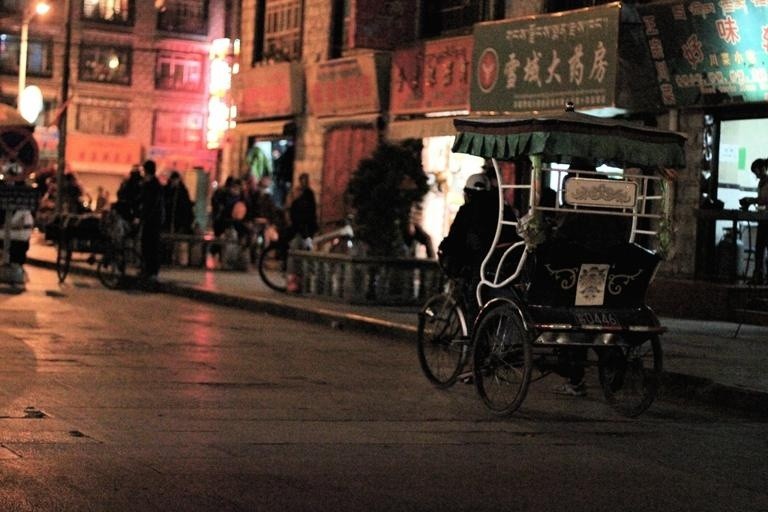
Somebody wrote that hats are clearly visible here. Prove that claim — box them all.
[466,174,491,192]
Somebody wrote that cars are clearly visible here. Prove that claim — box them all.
[0,207,37,267]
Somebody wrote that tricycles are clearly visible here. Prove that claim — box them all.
[409,99,692,421]
[42,208,133,294]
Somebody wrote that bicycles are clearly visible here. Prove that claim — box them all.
[255,212,312,293]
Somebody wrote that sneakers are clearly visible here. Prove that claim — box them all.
[550,377,587,395]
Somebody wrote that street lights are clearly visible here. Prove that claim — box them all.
[16,2,53,114]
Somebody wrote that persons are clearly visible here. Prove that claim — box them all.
[1,150,82,212]
[548,345,590,398]
[485,166,559,213]
[436,172,518,332]
[402,199,435,259]
[118,160,195,276]
[210,174,317,261]
[737,157,768,286]
